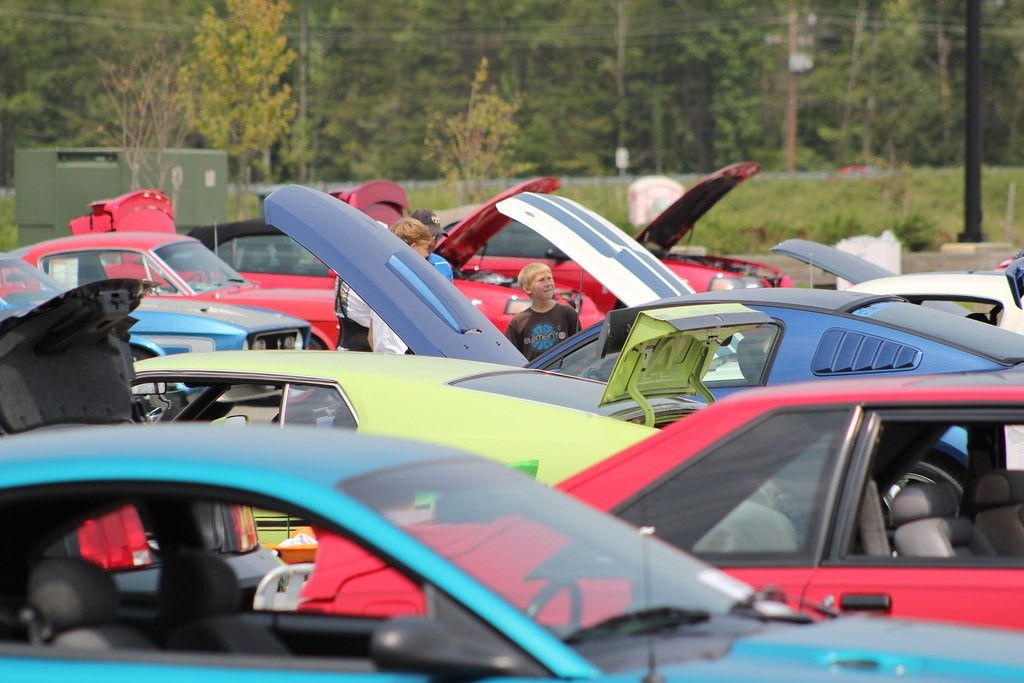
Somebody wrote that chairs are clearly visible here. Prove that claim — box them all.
[974,477,1024,555]
[737,331,774,383]
[151,544,292,659]
[889,483,997,564]
[21,551,158,654]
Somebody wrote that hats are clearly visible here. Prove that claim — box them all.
[411,208,448,238]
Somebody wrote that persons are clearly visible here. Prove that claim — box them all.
[503,262,583,363]
[332,218,390,352]
[367,215,433,356]
[409,206,454,287]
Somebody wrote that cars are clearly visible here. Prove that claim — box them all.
[0,158,1024,412]
[135,300,785,574]
[293,374,1024,636]
[1,424,1023,683]
